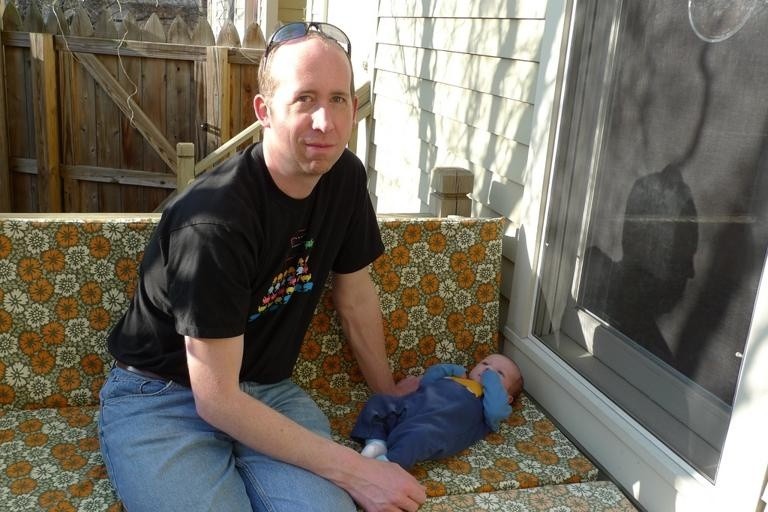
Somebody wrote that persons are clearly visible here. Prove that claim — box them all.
[98,22,428,512]
[350,352,524,470]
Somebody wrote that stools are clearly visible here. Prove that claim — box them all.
[408,479,640,512]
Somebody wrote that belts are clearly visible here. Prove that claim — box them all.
[117,361,162,379]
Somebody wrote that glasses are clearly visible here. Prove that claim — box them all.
[265,22,351,57]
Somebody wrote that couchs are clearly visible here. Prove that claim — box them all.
[1,215,600,512]
